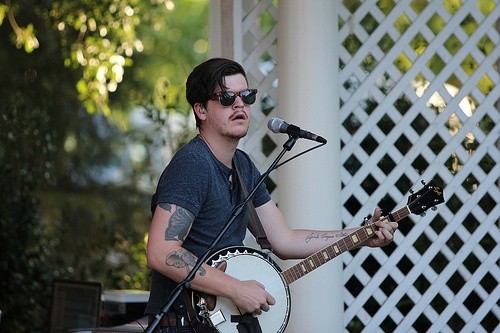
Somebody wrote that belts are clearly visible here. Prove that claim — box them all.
[148,314,191,327]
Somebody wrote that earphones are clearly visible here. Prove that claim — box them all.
[199,108,204,114]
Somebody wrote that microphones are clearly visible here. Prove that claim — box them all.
[267,117,327,143]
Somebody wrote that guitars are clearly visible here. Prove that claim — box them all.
[180,179,445,333]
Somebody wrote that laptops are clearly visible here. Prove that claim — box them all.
[47,279,101,333]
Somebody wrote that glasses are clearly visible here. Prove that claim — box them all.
[208,89,257,107]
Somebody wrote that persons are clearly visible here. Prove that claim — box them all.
[143,57,398,333]
